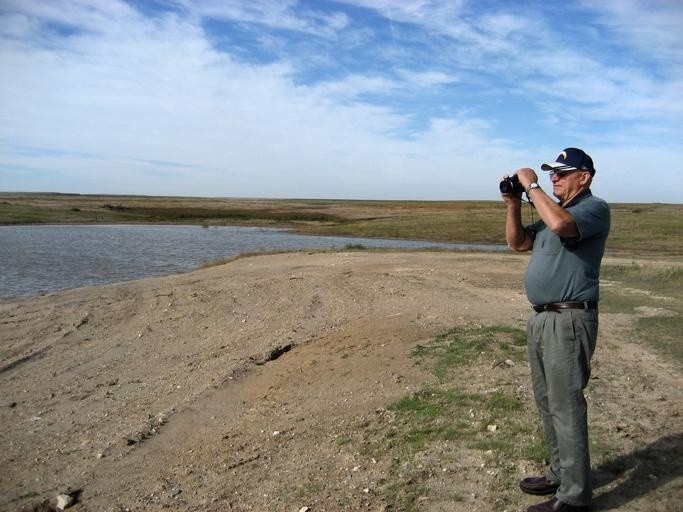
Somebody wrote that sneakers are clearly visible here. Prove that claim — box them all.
[527,497,593,512]
[519,475,561,495]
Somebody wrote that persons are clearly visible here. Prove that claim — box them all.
[501,148,612,511]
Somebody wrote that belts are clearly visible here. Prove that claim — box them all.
[530,301,598,312]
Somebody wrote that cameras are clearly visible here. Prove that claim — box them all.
[499,173,527,192]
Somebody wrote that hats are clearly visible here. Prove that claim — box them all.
[540,147,594,172]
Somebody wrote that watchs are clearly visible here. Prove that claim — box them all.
[526,181,541,200]
